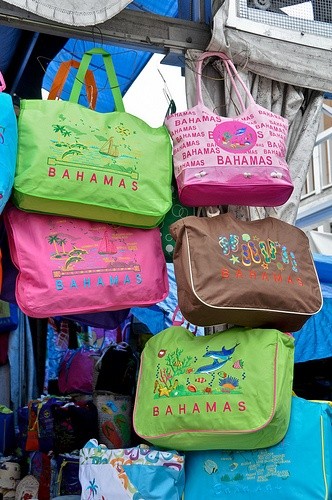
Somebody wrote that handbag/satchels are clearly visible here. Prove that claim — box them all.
[1,87,20,215]
[3,204,172,320]
[0,261,206,500]
[163,49,297,209]
[132,325,296,453]
[11,47,178,232]
[184,390,319,500]
[77,437,187,500]
[168,212,324,329]
